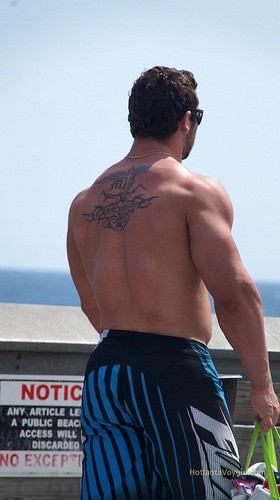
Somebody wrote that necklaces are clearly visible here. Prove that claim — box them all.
[126,151,173,158]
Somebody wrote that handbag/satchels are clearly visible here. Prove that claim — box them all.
[230,418,280,500]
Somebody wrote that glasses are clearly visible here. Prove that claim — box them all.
[187,107,203,125]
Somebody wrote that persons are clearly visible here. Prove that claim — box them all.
[65,66,280,500]
